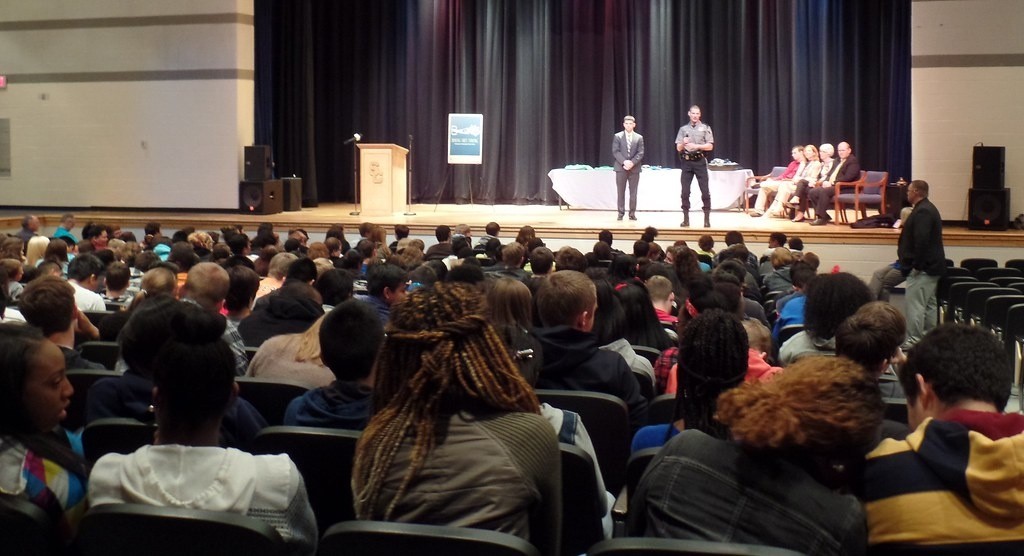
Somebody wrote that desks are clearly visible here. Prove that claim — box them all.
[547,168,755,210]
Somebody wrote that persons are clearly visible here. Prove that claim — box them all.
[898,180,945,351]
[354,282,561,556]
[612,116,644,220]
[0,322,84,536]
[870,208,912,302]
[860,325,1024,556]
[750,141,860,225]
[675,106,714,227]
[86,293,318,556]
[836,299,910,453]
[0,214,875,540]
[626,355,881,556]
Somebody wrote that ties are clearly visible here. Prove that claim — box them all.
[627,134,631,158]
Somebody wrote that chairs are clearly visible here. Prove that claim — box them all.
[924,258,1024,398]
[743,166,888,224]
[0,324,906,556]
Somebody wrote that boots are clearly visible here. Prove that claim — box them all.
[704,211,710,227]
[680,212,689,227]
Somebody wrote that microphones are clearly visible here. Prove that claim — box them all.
[344,133,363,146]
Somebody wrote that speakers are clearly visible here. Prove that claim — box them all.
[886,185,912,221]
[239,145,302,215]
[968,146,1010,232]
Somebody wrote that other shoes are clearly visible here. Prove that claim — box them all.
[618,216,623,220]
[790,215,805,223]
[900,343,911,350]
[629,216,637,220]
[750,212,762,217]
[827,214,832,223]
[769,210,786,218]
[809,215,827,225]
[784,202,800,209]
